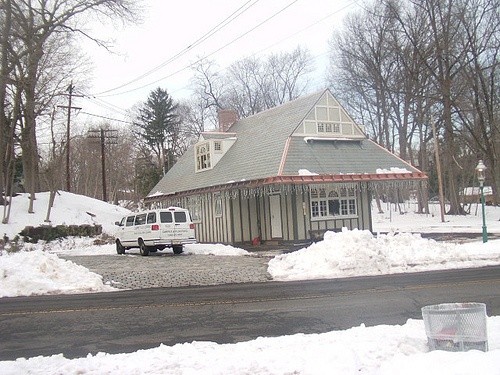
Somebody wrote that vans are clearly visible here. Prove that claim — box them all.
[114,207,195,255]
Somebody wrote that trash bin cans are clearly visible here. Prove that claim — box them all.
[421,301,490,353]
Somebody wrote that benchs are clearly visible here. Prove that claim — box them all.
[308,228,342,244]
